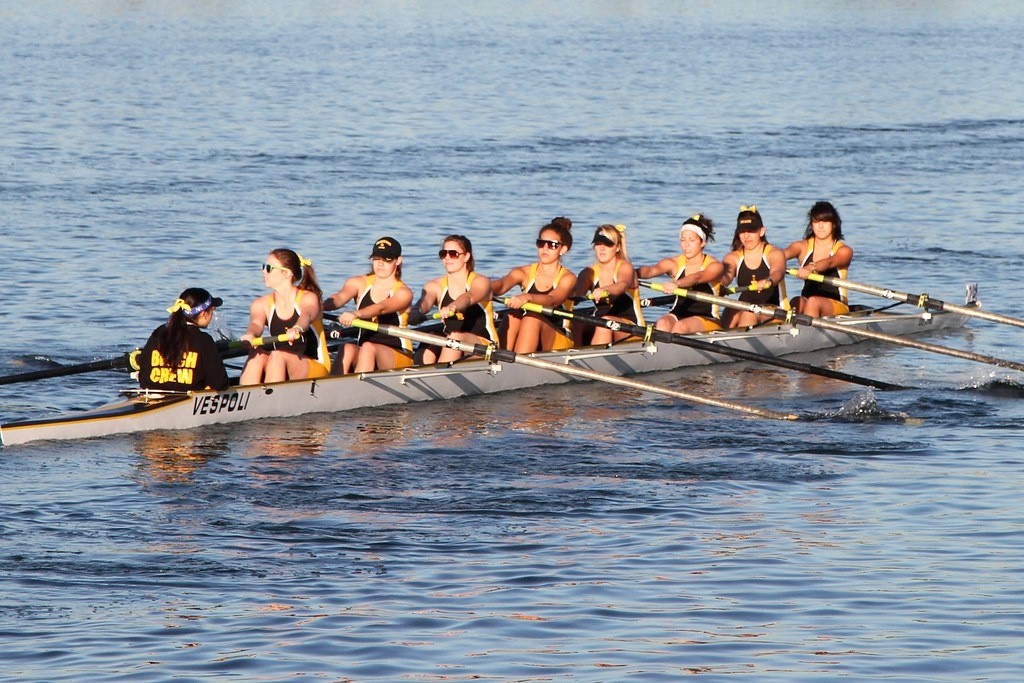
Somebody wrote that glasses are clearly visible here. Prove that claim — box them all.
[535,239,565,250]
[439,249,465,260]
[373,255,398,263]
[262,264,294,276]
[204,306,216,314]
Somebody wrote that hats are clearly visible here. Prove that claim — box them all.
[736,206,763,234]
[591,229,615,245]
[183,295,223,317]
[369,237,401,258]
[680,214,709,242]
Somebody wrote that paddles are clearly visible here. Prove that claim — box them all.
[784,266,1024,329]
[322,313,812,421]
[0,332,302,385]
[574,282,771,331]
[424,291,608,320]
[490,294,904,390]
[640,279,1024,372]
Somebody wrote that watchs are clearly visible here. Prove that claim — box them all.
[765,277,773,287]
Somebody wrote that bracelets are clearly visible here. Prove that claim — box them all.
[292,325,304,334]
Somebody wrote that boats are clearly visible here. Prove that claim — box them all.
[0,282,984,451]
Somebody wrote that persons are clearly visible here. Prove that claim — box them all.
[491,217,578,355]
[321,237,416,375]
[634,214,725,336]
[567,224,646,347]
[239,248,331,386]
[783,201,854,318]
[716,205,788,329]
[140,287,230,391]
[405,235,500,367]
